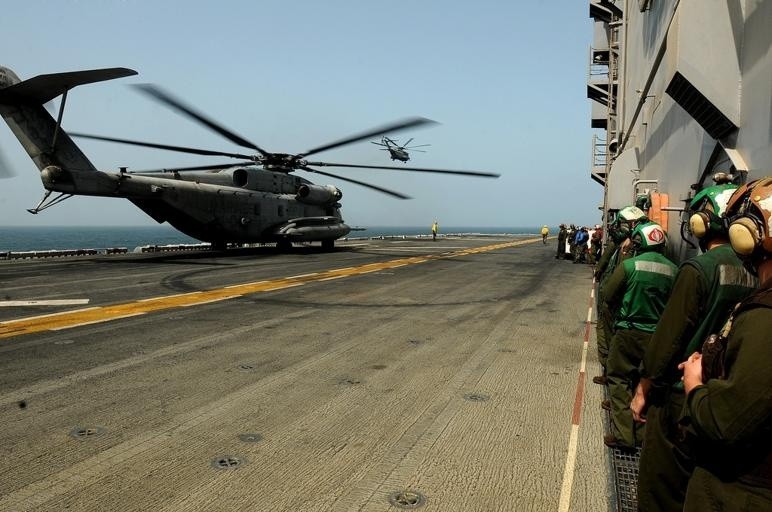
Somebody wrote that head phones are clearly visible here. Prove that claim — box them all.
[727,176,765,256]
[609,216,669,250]
[689,196,711,238]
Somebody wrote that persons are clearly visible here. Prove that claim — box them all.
[540,224,549,244]
[601,221,678,454]
[431,220,438,241]
[675,172,770,511]
[552,204,649,410]
[629,180,758,510]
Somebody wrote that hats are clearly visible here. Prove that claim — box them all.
[559,223,575,228]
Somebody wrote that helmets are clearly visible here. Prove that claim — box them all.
[721,175,772,264]
[630,221,667,253]
[595,224,601,229]
[614,206,648,237]
[687,182,740,247]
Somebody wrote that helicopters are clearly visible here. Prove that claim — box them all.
[0,64,502,249]
[370,134,432,164]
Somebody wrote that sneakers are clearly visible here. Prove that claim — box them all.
[603,434,636,451]
[592,375,609,385]
[601,399,611,411]
